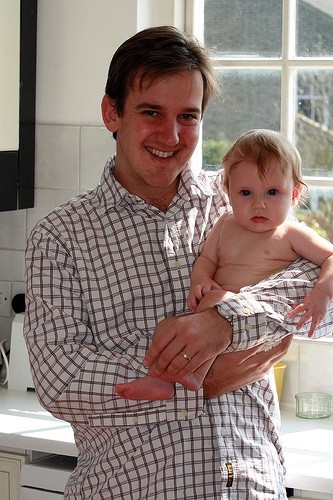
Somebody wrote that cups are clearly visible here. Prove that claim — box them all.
[295,392,333,419]
[273,362,288,403]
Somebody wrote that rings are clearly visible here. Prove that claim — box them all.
[180,352,192,361]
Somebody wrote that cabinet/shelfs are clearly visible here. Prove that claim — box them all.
[0,384,333,500]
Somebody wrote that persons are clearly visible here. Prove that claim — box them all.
[24,26,333,500]
[115,127,333,401]
